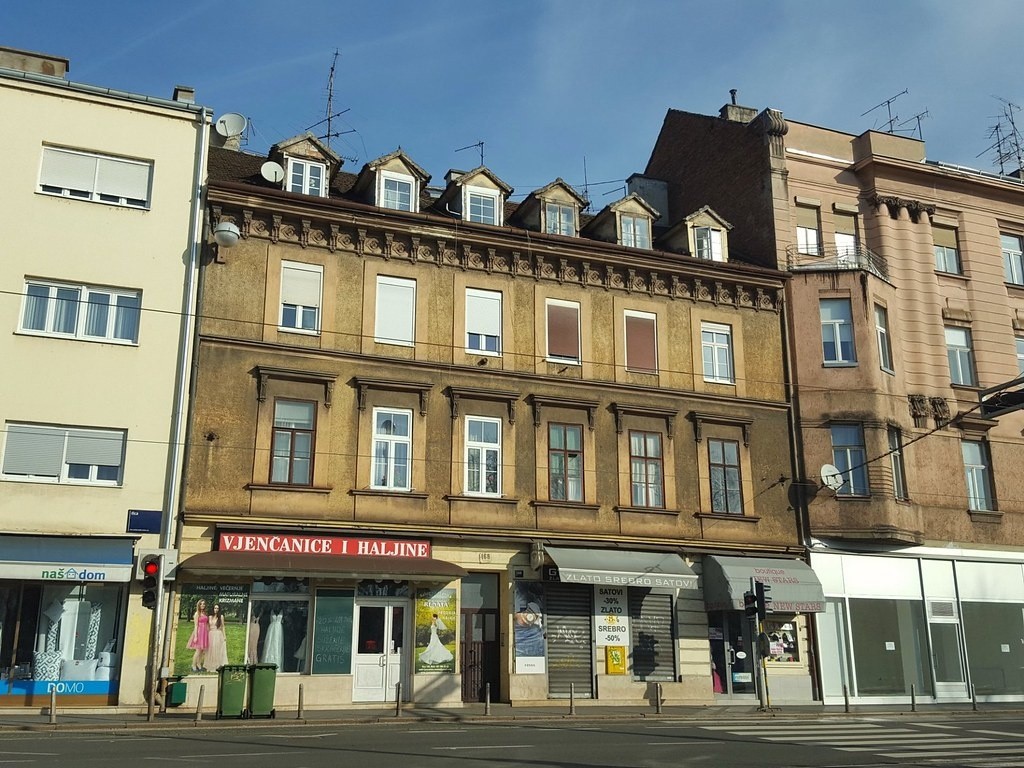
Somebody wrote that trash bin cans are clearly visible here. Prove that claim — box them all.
[247,663,278,719]
[215,664,247,720]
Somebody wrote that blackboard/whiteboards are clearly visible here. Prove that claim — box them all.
[311,586,354,676]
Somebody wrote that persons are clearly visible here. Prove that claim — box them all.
[248,600,260,664]
[262,608,284,673]
[202,603,229,673]
[418,614,453,664]
[294,616,308,672]
[186,599,209,672]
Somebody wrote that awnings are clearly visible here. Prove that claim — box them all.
[702,555,826,613]
[180,550,469,583]
[543,546,699,589]
[0,533,142,583]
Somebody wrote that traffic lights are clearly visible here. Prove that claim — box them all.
[142,559,160,607]
[744,590,758,619]
[755,582,773,622]
